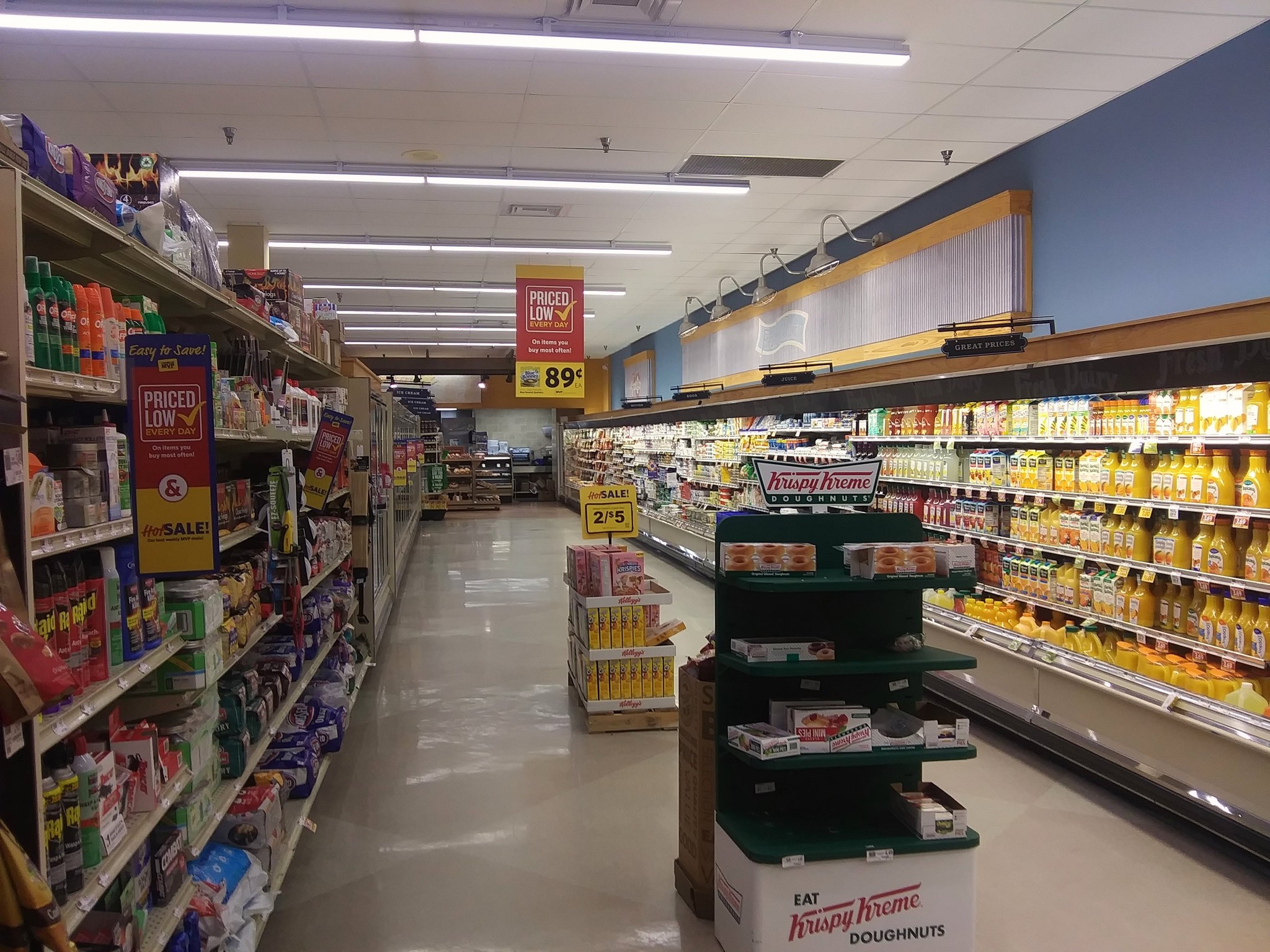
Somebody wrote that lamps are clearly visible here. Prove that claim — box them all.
[389,374,397,389]
[168,158,750,199]
[804,215,884,282]
[334,306,594,319]
[213,231,672,255]
[413,374,422,382]
[505,374,513,383]
[750,253,806,308]
[477,375,489,390]
[0,4,909,68]
[300,277,626,299]
[678,298,712,338]
[710,276,754,324]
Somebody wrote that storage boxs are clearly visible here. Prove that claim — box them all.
[521,482,529,492]
[677,657,714,890]
[566,543,675,700]
[222,267,343,369]
[546,480,554,490]
[538,492,554,501]
[719,537,976,841]
[536,480,545,488]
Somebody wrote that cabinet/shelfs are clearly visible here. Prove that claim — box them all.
[0,166,369,951]
[560,434,1270,749]
[475,458,513,496]
[441,459,473,505]
[714,511,982,864]
[562,574,677,715]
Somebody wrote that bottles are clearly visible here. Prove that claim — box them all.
[20,255,144,381]
[269,371,323,435]
[876,378,1270,722]
[39,734,102,907]
[33,543,163,720]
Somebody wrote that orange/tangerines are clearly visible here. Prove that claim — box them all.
[1004,472,1270,618]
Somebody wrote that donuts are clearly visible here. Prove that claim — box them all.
[808,642,834,660]
[875,547,935,573]
[725,545,815,571]
